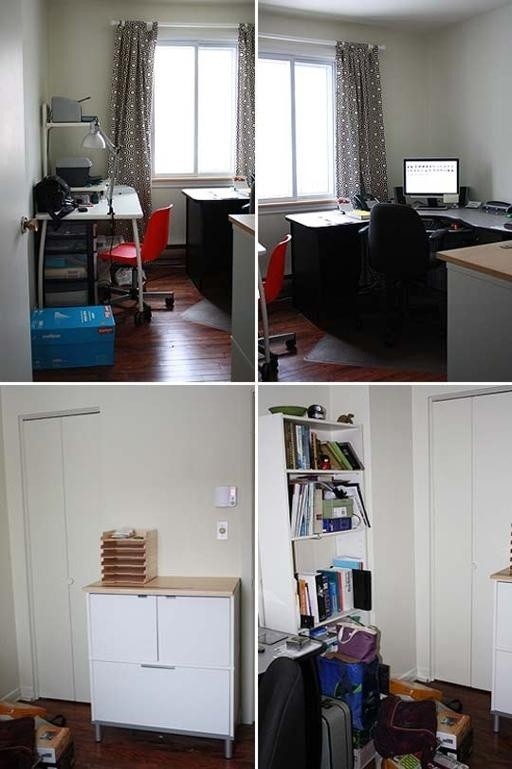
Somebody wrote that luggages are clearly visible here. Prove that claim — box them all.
[319,693,355,769]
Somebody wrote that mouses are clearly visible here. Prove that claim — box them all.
[451,223,462,230]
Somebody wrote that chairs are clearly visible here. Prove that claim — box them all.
[257,232,297,356]
[357,203,475,347]
[97,204,176,327]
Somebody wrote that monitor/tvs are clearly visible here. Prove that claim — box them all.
[402,157,460,210]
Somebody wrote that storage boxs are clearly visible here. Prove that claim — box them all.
[30,306,116,370]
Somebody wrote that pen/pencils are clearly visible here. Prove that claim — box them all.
[78,205,93,207]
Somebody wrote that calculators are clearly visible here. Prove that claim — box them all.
[465,200,482,208]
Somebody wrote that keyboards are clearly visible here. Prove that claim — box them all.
[422,222,447,230]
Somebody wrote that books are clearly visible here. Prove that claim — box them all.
[284,420,372,629]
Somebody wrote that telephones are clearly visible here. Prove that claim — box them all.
[354,193,380,210]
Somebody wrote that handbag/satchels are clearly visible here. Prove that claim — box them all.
[35,178,70,212]
[316,651,380,731]
[33,712,68,730]
[373,695,437,758]
[335,619,379,664]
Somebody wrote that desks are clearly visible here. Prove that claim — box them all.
[258,243,278,377]
[285,200,512,327]
[181,186,252,312]
[34,183,146,327]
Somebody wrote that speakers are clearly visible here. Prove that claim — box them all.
[460,187,469,206]
[395,187,406,204]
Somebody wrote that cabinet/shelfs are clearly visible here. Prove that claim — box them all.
[489,565,512,734]
[85,578,243,759]
[254,410,376,654]
